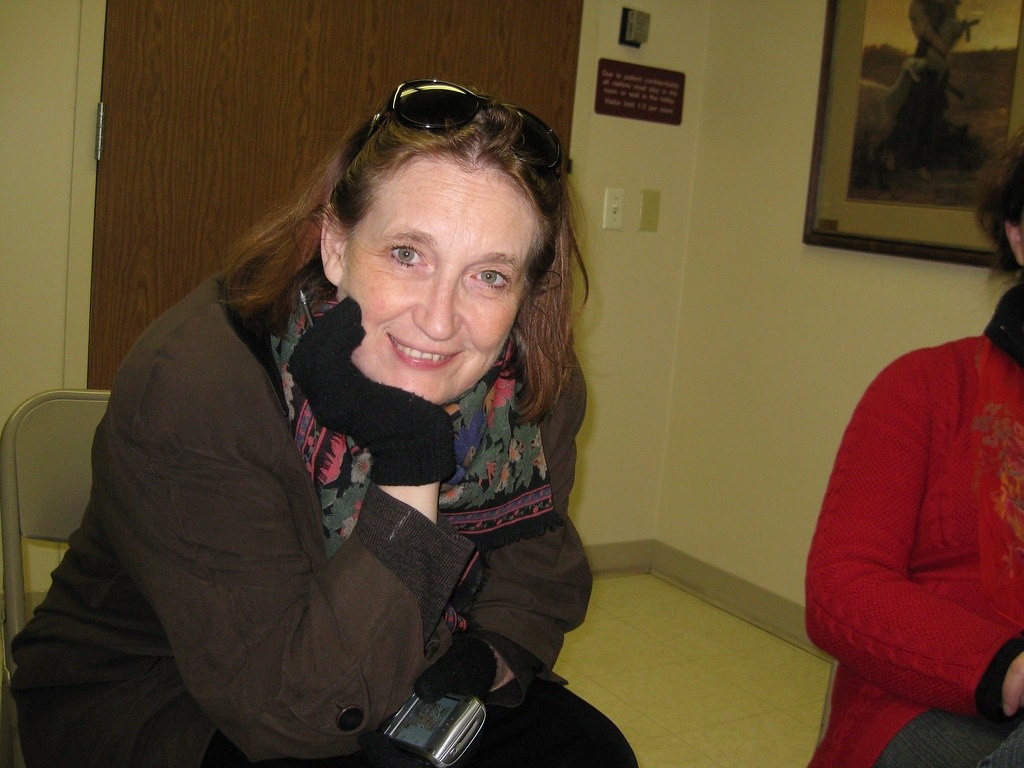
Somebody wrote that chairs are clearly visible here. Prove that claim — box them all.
[0,387,118,766]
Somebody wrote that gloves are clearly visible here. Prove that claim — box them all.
[415,635,497,705]
[288,295,459,486]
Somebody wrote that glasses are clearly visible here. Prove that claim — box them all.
[365,80,565,182]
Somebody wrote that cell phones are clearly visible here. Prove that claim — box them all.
[378,690,486,768]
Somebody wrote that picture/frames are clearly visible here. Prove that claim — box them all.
[802,0,1024,265]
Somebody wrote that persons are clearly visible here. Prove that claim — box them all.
[9,78,638,767]
[806,132,1024,767]
